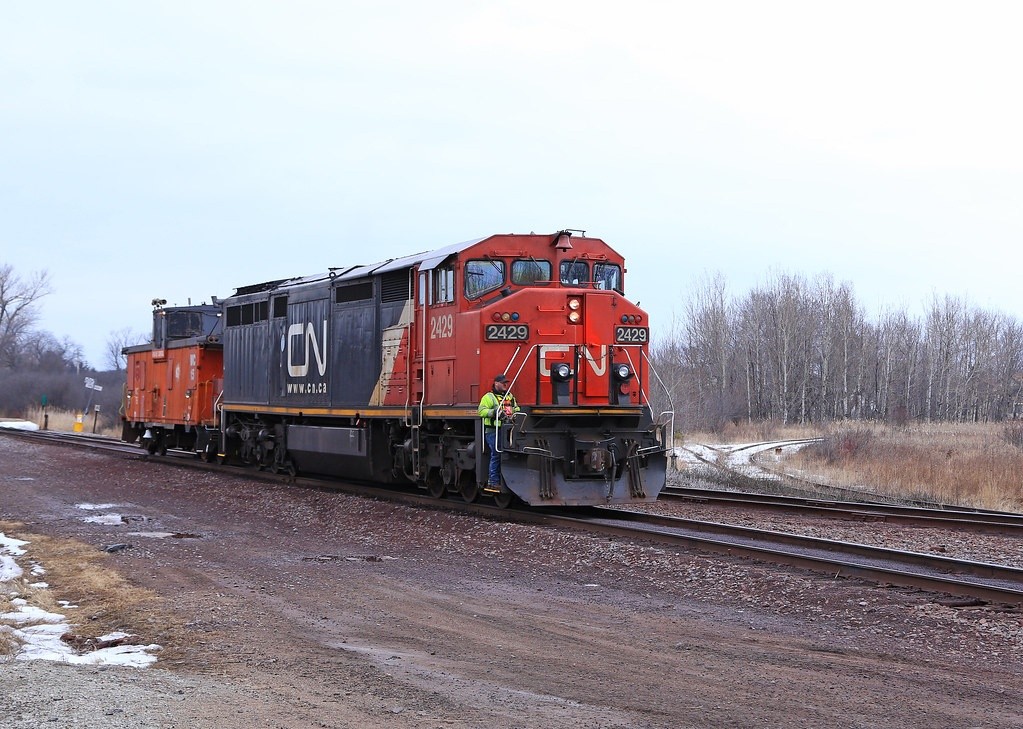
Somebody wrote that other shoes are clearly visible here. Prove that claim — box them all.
[487,484,502,490]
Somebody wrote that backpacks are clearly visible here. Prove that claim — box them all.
[493,410,506,420]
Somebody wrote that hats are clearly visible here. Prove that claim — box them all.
[495,375,510,383]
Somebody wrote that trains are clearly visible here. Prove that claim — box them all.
[119,229,674,509]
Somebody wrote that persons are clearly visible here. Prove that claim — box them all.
[477,375,520,489]
